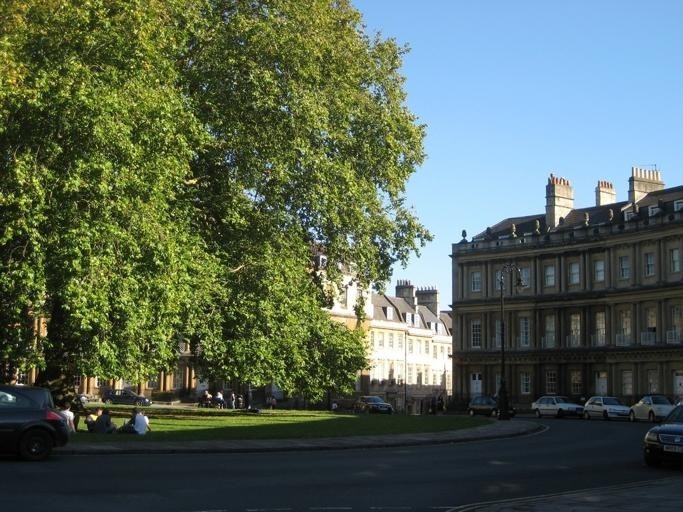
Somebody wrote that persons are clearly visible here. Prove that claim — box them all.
[199,388,249,409]
[272,395,277,409]
[96,407,117,433]
[331,400,338,410]
[118,406,152,434]
[86,408,103,432]
[60,401,77,433]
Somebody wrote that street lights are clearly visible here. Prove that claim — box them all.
[404,330,409,415]
[499,264,529,423]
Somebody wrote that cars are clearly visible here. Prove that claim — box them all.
[467,395,517,417]
[102,389,152,406]
[0,386,68,461]
[530,395,683,467]
[353,395,394,413]
